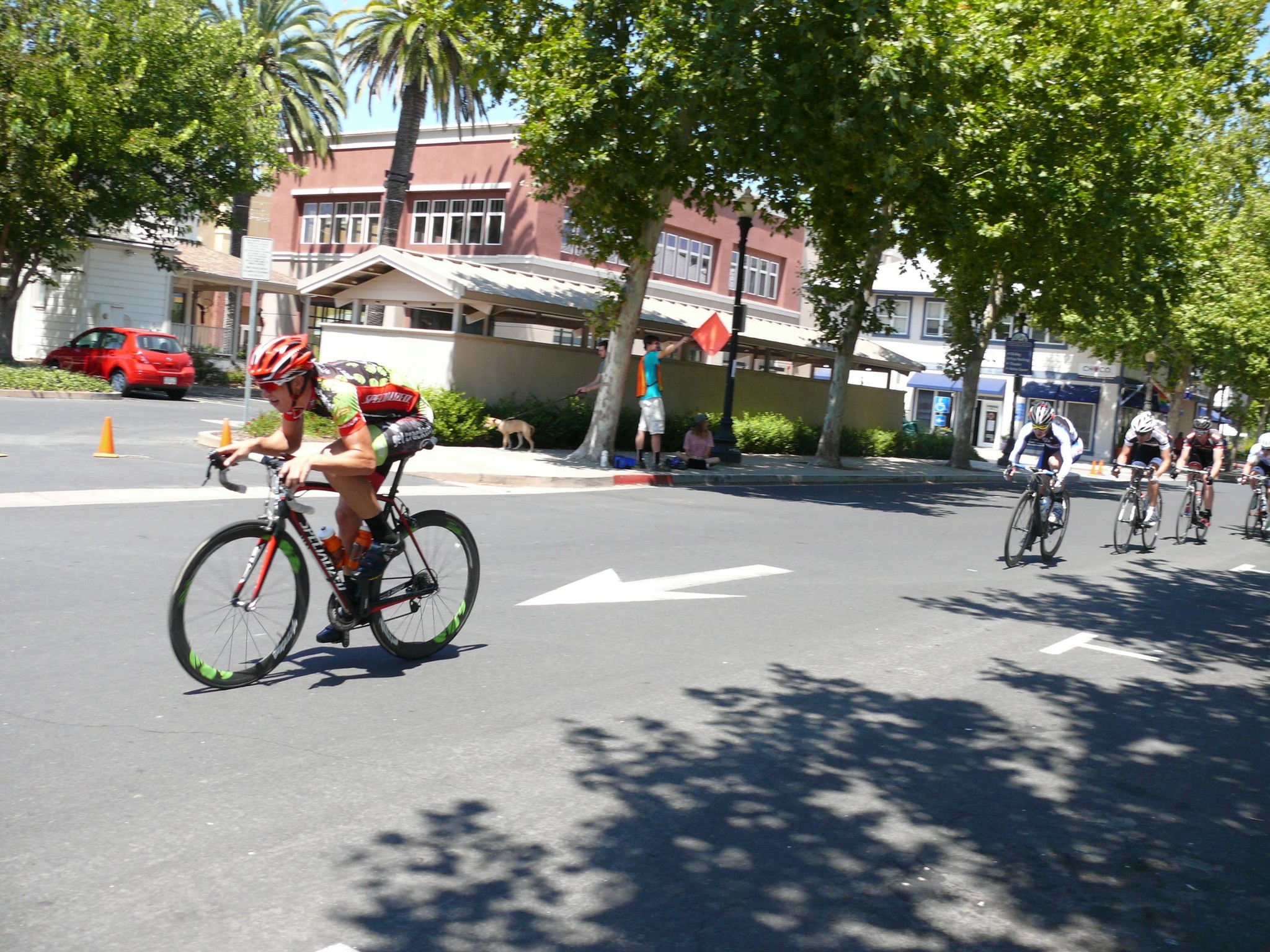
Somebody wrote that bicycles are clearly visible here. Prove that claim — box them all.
[1002,461,1071,568]
[1168,460,1211,545]
[165,453,481,690]
[1237,473,1270,540]
[1110,458,1164,554]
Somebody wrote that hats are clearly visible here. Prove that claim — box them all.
[691,413,707,426]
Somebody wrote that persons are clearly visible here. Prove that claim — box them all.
[207,333,434,644]
[574,340,608,393]
[1170,416,1225,526]
[1173,432,1184,460]
[635,335,691,472]
[1003,400,1083,550]
[1241,432,1270,532]
[1110,411,1171,525]
[676,414,720,470]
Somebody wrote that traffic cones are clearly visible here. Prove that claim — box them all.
[1087,460,1098,476]
[92,416,119,458]
[1095,459,1105,475]
[997,301,1028,468]
[218,417,232,448]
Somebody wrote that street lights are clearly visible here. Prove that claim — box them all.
[1141,350,1157,414]
[707,183,758,468]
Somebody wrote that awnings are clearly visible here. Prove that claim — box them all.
[1119,388,1170,414]
[1019,381,1100,404]
[814,368,832,380]
[906,372,1006,395]
[1199,407,1236,425]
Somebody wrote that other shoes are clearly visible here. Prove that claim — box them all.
[1264,518,1270,531]
[652,462,670,471]
[1251,494,1258,509]
[634,461,646,469]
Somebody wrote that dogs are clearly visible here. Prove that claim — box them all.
[484,418,534,452]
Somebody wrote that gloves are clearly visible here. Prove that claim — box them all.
[1168,470,1179,479]
[1205,477,1213,485]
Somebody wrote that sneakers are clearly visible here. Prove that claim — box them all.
[317,610,356,643]
[1200,517,1210,527]
[1048,503,1064,525]
[350,537,406,581]
[1185,504,1192,516]
[1020,530,1029,547]
[1143,510,1158,524]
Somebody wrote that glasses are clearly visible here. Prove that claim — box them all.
[1032,423,1049,431]
[254,372,307,393]
[1134,433,1148,436]
[1261,448,1270,452]
[1194,428,1207,435]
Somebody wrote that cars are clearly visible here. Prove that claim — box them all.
[41,326,196,402]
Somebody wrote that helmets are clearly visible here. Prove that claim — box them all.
[1130,410,1156,433]
[1258,433,1270,447]
[248,334,318,379]
[1029,402,1055,426]
[1194,416,1212,429]
